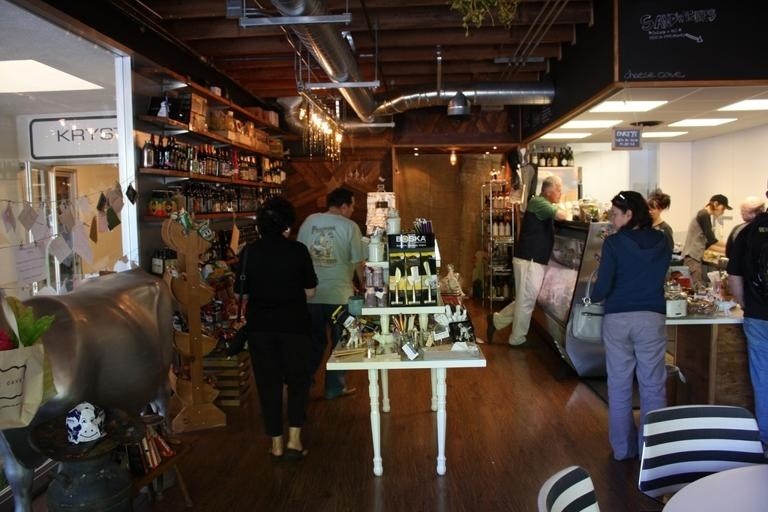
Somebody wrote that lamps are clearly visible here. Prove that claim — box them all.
[290,37,347,170]
[448,89,472,117]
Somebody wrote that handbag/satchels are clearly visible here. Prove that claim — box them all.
[572,268,604,343]
[0,297,46,431]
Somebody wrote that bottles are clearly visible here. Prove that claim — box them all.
[212,225,256,248]
[143,134,282,183]
[531,144,573,167]
[492,192,511,209]
[153,247,177,270]
[369,237,385,262]
[492,221,511,237]
[185,183,281,213]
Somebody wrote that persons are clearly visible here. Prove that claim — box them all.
[295,188,368,398]
[485,176,570,349]
[724,197,766,308]
[233,198,319,463]
[645,190,675,252]
[725,196,768,459]
[681,194,733,286]
[592,190,672,467]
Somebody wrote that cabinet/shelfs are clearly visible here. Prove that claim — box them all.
[480,176,515,304]
[158,219,227,435]
[143,72,292,407]
[534,215,616,381]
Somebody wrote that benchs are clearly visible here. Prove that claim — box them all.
[27,439,194,511]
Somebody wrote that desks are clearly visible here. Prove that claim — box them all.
[324,288,489,478]
[659,463,768,512]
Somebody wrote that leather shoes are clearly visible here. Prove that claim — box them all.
[487,314,496,342]
[509,340,536,348]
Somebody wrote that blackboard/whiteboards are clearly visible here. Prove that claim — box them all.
[610,126,644,151]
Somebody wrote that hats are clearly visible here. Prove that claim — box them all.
[710,195,732,210]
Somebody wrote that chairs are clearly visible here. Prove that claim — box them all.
[638,404,764,511]
[536,464,601,511]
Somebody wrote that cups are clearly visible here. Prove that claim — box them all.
[387,218,401,235]
[348,296,362,316]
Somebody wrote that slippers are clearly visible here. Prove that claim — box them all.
[271,448,309,462]
